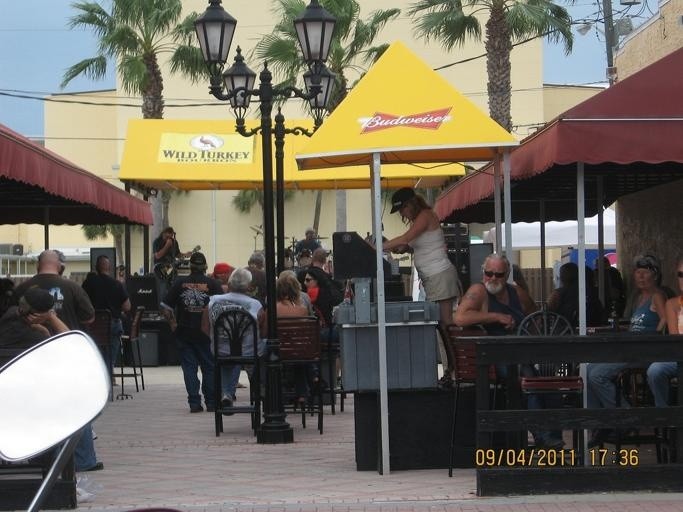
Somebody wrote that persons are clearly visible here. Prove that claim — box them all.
[372,186,462,386]
[647,260,683,409]
[304,267,341,386]
[152,227,193,314]
[78,255,132,373]
[454,253,580,459]
[160,252,224,413]
[586,258,667,450]
[257,270,309,408]
[202,268,266,413]
[545,262,579,334]
[295,229,323,258]
[11,249,95,335]
[0,286,103,472]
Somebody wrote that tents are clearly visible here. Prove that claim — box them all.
[1,125,151,377]
[434,48,683,467]
[294,39,519,479]
[118,116,466,307]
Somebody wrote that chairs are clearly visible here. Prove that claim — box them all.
[212,309,261,436]
[518,310,585,458]
[112,306,146,394]
[444,323,505,487]
[268,315,325,431]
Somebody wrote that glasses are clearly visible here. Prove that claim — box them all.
[305,278,314,281]
[301,248,312,255]
[636,259,657,271]
[678,271,683,277]
[484,269,507,277]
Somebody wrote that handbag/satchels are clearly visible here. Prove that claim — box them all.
[301,291,319,320]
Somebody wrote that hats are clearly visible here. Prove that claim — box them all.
[214,264,227,272]
[560,263,578,280]
[596,257,610,271]
[25,287,55,311]
[190,252,206,265]
[390,187,415,214]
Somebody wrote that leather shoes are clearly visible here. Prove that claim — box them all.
[86,462,103,470]
[587,437,601,450]
[208,406,217,411]
[191,407,203,412]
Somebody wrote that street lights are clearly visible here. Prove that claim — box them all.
[576,19,621,87]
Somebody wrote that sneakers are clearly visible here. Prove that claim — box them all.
[91,431,96,439]
[258,385,265,398]
[312,376,319,384]
[296,397,304,408]
[222,394,234,416]
[235,381,246,388]
[552,448,573,466]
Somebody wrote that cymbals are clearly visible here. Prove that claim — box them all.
[274,235,290,240]
[310,235,330,239]
[248,223,266,236]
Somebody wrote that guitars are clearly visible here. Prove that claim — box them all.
[152,245,201,285]
